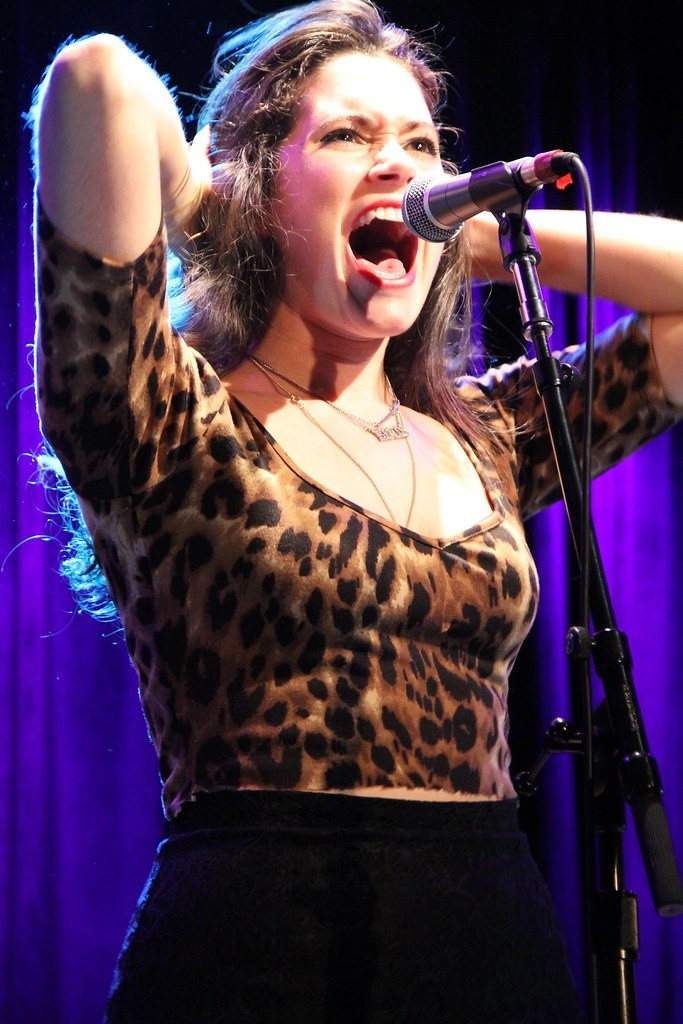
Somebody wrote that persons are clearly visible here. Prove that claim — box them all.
[30,0,683,1024]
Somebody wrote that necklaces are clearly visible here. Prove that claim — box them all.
[247,350,415,532]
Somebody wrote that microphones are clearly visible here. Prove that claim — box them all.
[401,147,580,244]
